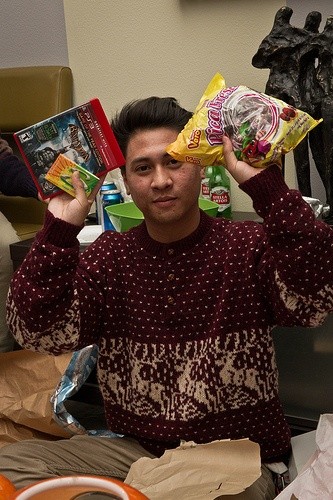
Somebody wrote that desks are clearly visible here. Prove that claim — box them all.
[10,211,333,432]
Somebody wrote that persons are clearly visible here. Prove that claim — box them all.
[0,137,50,353]
[0,96,333,500]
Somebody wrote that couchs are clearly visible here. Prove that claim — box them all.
[0,66,74,273]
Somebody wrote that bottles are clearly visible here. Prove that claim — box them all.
[200,165,213,199]
[209,165,232,219]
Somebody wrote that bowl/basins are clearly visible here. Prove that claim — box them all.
[0,475,151,500]
[105,197,220,233]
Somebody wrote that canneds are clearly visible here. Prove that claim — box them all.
[100,190,124,231]
[96,181,117,224]
